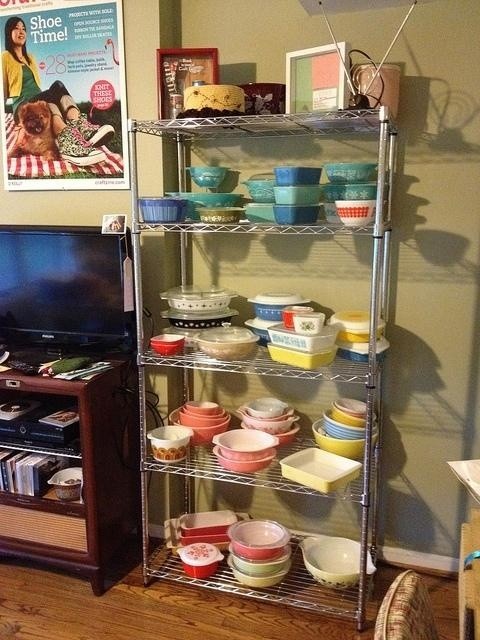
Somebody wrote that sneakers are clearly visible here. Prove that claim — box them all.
[66,115,115,148]
[58,127,107,166]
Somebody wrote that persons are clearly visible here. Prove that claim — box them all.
[1,17,116,166]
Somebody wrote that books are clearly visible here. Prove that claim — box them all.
[38,405,80,428]
[36,356,114,381]
[446,459,479,506]
[0,350,13,373]
[0,441,72,499]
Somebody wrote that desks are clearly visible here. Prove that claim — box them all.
[445,453,480,639]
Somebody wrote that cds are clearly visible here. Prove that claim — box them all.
[0,399,41,421]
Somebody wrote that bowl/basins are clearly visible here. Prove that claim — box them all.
[323,163,378,184]
[150,341,185,356]
[300,536,377,588]
[237,408,300,434]
[167,192,244,220]
[177,520,231,546]
[185,167,231,188]
[227,556,291,588]
[325,310,386,342]
[228,520,292,560]
[331,402,376,427]
[228,543,291,575]
[274,187,322,205]
[337,336,390,362]
[179,407,229,427]
[267,342,338,369]
[240,422,299,445]
[169,408,230,446]
[274,166,321,185]
[273,204,320,225]
[48,467,82,501]
[280,447,362,493]
[183,406,225,418]
[335,200,375,226]
[147,425,193,464]
[165,512,252,555]
[293,312,326,335]
[180,510,238,535]
[312,418,379,460]
[213,446,277,472]
[335,397,367,418]
[186,401,219,415]
[139,198,187,223]
[322,182,376,202]
[323,202,341,224]
[281,306,314,329]
[151,334,185,345]
[244,398,289,417]
[267,323,337,352]
[239,407,295,421]
[323,410,378,440]
[244,203,273,223]
[212,429,279,461]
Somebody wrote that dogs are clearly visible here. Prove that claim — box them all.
[7,100,58,163]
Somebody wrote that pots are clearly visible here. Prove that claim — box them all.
[196,206,246,223]
[242,173,275,203]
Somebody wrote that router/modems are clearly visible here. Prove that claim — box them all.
[318,0,419,117]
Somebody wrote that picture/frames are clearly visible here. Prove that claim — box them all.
[286,43,346,116]
[1,0,129,191]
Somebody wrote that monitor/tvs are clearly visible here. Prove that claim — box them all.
[1,224,132,367]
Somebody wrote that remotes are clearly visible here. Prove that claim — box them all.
[7,360,39,375]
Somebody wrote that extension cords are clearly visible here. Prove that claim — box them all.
[103,352,135,361]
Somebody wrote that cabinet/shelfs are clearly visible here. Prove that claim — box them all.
[0,362,143,598]
[125,106,397,632]
